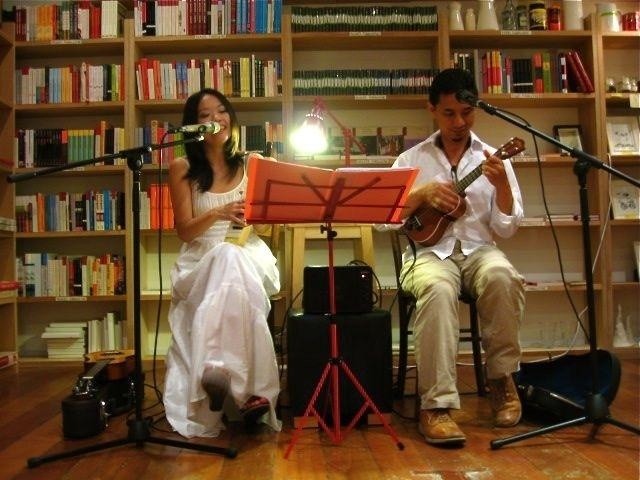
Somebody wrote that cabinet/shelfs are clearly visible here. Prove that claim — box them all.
[445,1,596,361]
[598,0,640,355]
[288,2,447,333]
[0,2,129,371]
[130,1,290,373]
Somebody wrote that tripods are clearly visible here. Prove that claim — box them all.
[283,219,405,459]
[490,108,640,450]
[6,134,238,467]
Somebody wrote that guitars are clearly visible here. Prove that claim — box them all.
[403,136,525,246]
[72,349,136,399]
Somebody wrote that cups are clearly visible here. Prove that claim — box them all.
[623,12,640,30]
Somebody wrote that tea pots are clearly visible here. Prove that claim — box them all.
[594,1,621,32]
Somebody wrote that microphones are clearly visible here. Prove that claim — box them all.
[168,121,220,134]
[455,89,496,115]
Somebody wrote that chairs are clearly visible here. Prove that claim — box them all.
[390,226,486,397]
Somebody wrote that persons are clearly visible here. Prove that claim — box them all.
[372,68,526,443]
[162,88,282,442]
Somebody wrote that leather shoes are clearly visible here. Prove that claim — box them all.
[418,409,466,443]
[487,374,522,426]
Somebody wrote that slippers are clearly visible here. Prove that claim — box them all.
[202,367,270,418]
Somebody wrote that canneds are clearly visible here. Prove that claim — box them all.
[517,3,561,31]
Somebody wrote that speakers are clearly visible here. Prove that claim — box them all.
[303,265,373,313]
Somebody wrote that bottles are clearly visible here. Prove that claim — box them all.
[501,0,516,31]
[465,8,476,30]
[515,5,529,31]
[223,140,275,246]
[446,1,465,30]
[604,75,638,93]
[562,1,584,31]
[545,5,561,31]
[530,2,546,31]
[475,0,501,30]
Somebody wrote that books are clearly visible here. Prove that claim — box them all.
[16,190,125,232]
[2,218,18,232]
[132,0,283,227]
[291,7,439,159]
[13,1,124,41]
[2,351,17,366]
[14,252,124,297]
[14,120,124,168]
[41,312,124,359]
[450,48,594,93]
[15,62,124,104]
[2,280,19,298]
